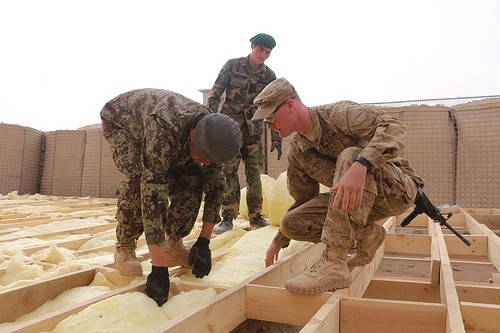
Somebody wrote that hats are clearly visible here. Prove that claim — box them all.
[250,33,276,49]
[251,78,297,121]
[194,113,243,163]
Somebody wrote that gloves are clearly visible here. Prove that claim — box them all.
[188,237,212,278]
[270,140,283,160]
[144,266,171,306]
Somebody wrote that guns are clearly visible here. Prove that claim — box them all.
[400,192,472,247]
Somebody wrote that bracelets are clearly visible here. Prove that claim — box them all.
[353,156,372,174]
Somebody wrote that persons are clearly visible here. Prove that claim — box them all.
[100,88,243,308]
[206,33,283,234]
[250,77,426,297]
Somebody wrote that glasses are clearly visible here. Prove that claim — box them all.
[262,111,278,123]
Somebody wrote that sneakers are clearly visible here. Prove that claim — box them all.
[163,237,194,268]
[285,245,352,294]
[114,243,145,276]
[214,218,233,234]
[348,224,385,266]
[249,217,269,227]
